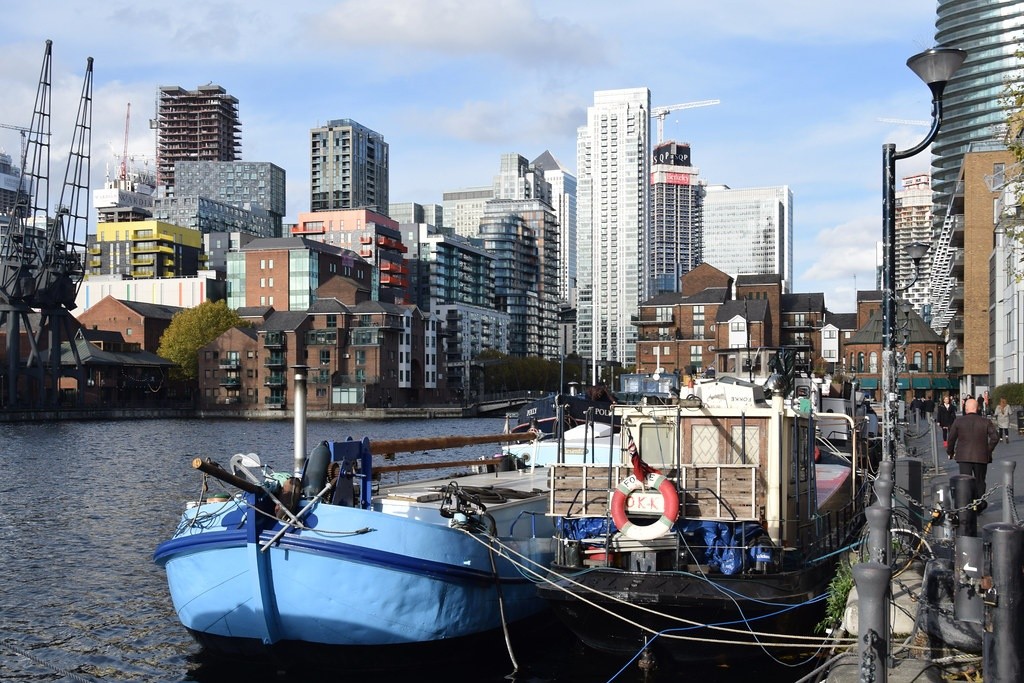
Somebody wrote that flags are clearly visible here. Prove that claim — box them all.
[627,435,662,485]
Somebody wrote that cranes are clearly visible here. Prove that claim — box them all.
[0,123,52,193]
[114,154,158,165]
[650,98,719,145]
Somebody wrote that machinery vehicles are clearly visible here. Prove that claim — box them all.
[121,102,132,179]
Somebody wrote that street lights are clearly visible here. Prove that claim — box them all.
[877,45,972,410]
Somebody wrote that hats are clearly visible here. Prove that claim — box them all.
[969,395,975,399]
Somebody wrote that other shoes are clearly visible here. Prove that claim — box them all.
[1006,439,1009,444]
[1000,440,1003,443]
[977,500,987,514]
[944,441,948,447]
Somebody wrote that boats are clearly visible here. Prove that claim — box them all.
[540,341,867,663]
[148,452,577,659]
[500,384,624,468]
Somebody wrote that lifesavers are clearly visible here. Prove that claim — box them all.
[609,471,679,542]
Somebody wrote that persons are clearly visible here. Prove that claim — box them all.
[950,394,984,419]
[946,399,999,515]
[910,395,935,427]
[937,395,956,448]
[995,398,1013,444]
